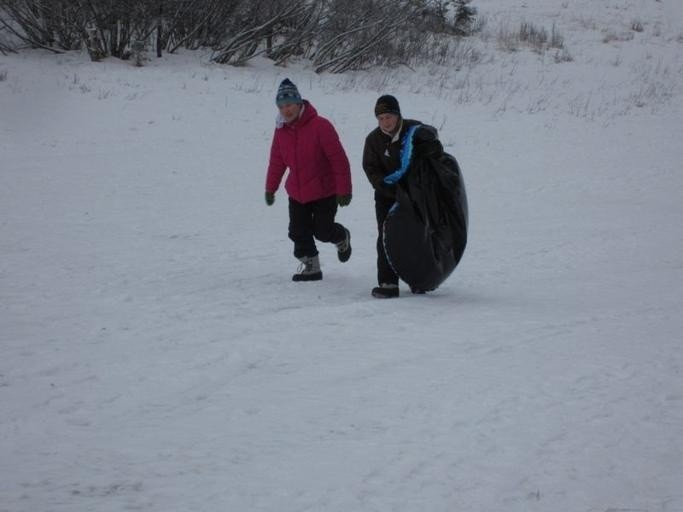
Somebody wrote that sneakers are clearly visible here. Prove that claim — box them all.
[371,283,399,298]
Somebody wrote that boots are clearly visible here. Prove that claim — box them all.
[292,252,322,281]
[334,228,351,262]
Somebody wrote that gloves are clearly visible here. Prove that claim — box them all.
[264,191,274,206]
[335,195,352,207]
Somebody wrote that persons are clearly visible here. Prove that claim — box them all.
[361,94,425,298]
[262,77,352,282]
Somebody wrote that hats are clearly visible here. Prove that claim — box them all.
[375,95,400,117]
[275,78,304,105]
